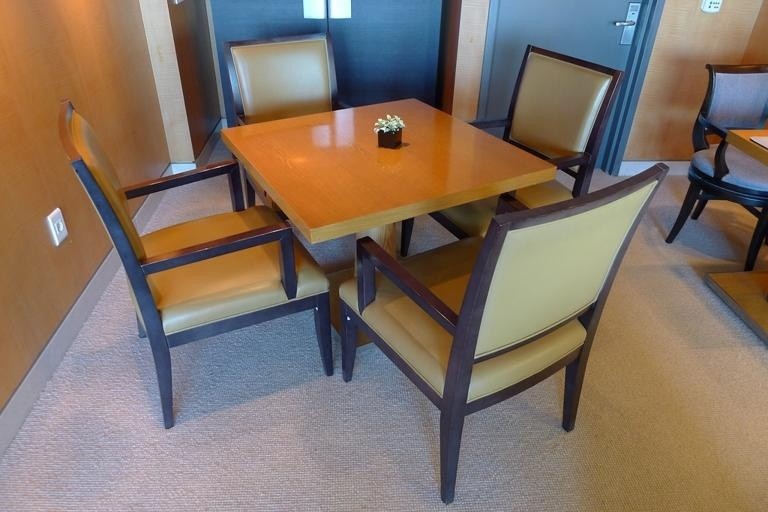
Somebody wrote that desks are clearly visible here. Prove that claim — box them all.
[221,96,558,348]
[704,130,767,346]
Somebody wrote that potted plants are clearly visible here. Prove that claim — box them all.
[372,113,405,149]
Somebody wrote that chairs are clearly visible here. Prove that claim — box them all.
[221,29,350,211]
[396,43,622,259]
[337,161,669,508]
[665,63,768,272]
[59,97,337,432]
